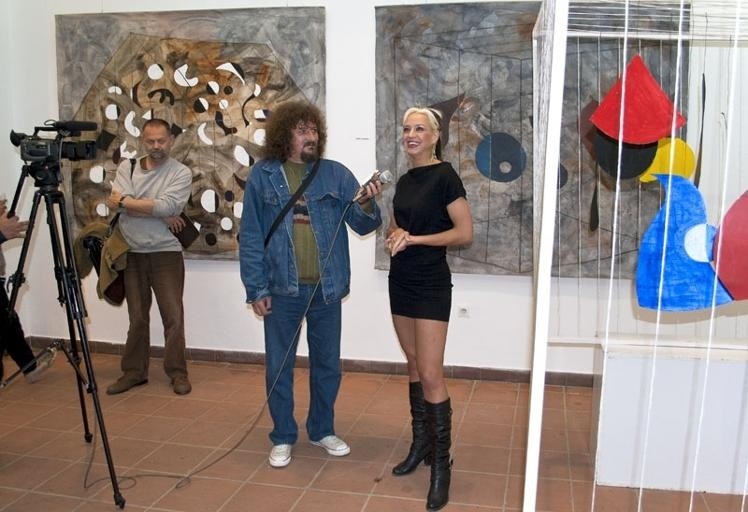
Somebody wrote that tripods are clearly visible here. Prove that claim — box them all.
[0,159,126,508]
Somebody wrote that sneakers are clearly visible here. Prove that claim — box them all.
[106,372,148,394]
[309,434,351,457]
[23,346,58,384]
[268,443,293,468]
[171,374,192,395]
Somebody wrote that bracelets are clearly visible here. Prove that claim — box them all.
[118,196,125,208]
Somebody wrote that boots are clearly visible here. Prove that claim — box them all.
[423,397,453,512]
[392,379,432,475]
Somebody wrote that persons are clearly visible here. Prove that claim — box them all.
[383,106,474,512]
[238,102,382,468]
[106,119,192,395]
[0,199,57,385]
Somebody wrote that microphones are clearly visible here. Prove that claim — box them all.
[351,170,394,203]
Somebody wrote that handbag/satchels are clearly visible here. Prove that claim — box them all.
[83,234,125,306]
